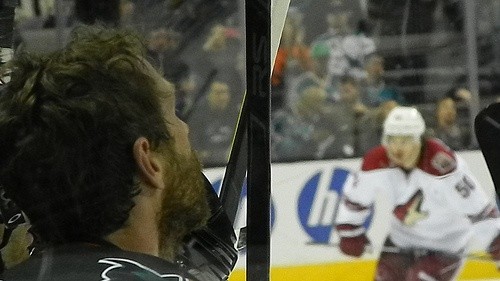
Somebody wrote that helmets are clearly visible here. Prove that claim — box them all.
[381,106,425,148]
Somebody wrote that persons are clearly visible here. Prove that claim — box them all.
[0,25,241,281]
[146,11,500,281]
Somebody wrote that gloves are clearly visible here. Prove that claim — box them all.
[336,224,370,257]
